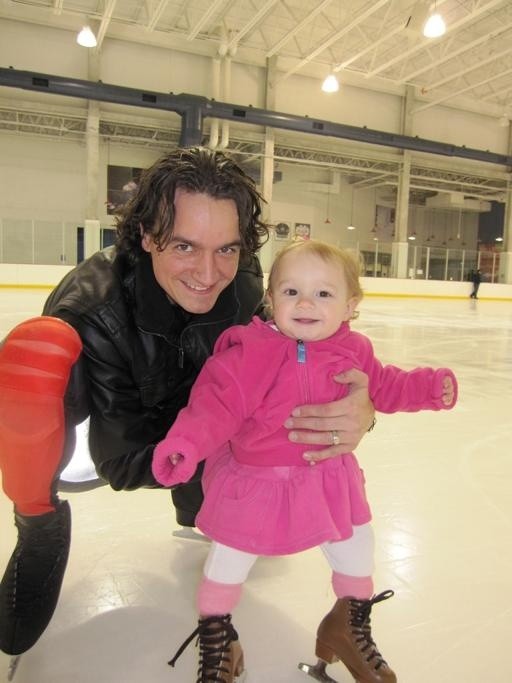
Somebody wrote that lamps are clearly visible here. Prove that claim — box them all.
[323,187,468,245]
[497,109,510,128]
[123,168,139,191]
[321,65,341,94]
[422,1,448,39]
[77,16,99,49]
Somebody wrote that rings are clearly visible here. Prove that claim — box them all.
[332,431,339,446]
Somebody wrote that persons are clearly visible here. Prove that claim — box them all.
[469,269,482,299]
[1,145,375,657]
[150,239,458,682]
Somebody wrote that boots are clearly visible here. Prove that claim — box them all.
[315,590,397,683]
[171,483,205,527]
[1,498,71,656]
[197,616,244,683]
